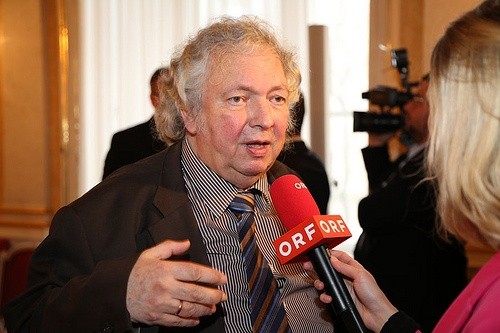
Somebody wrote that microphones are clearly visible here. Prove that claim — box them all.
[270,174,369,333]
